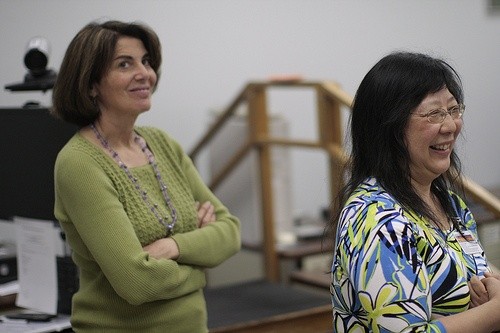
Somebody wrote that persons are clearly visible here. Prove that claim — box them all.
[320,50,500,333]
[52,19,242,333]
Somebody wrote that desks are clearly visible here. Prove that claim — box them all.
[204,277,337,332]
[242,186,500,275]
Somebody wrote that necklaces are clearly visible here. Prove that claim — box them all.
[86,122,179,230]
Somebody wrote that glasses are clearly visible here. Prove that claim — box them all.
[409,104,466,123]
